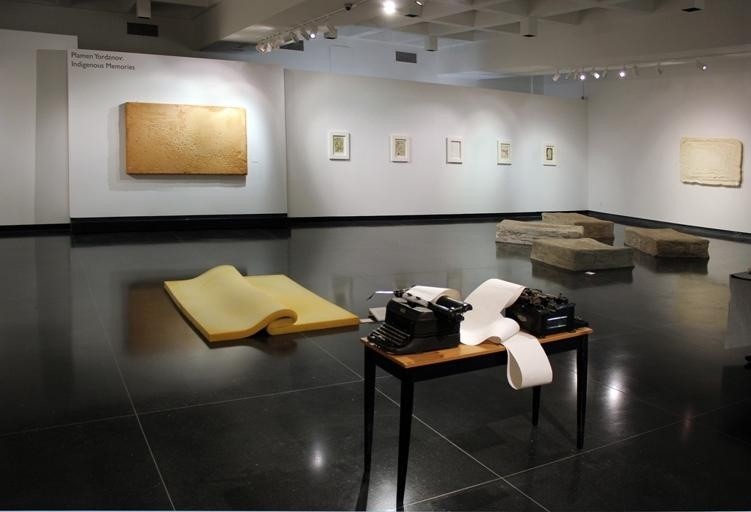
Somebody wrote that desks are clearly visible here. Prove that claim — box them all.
[360,325,595,503]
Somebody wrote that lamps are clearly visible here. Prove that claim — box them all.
[551,60,707,83]
[255,9,347,56]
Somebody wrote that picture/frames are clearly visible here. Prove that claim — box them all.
[496,140,512,165]
[390,134,409,162]
[543,144,558,166]
[329,131,350,160]
[446,136,463,163]
